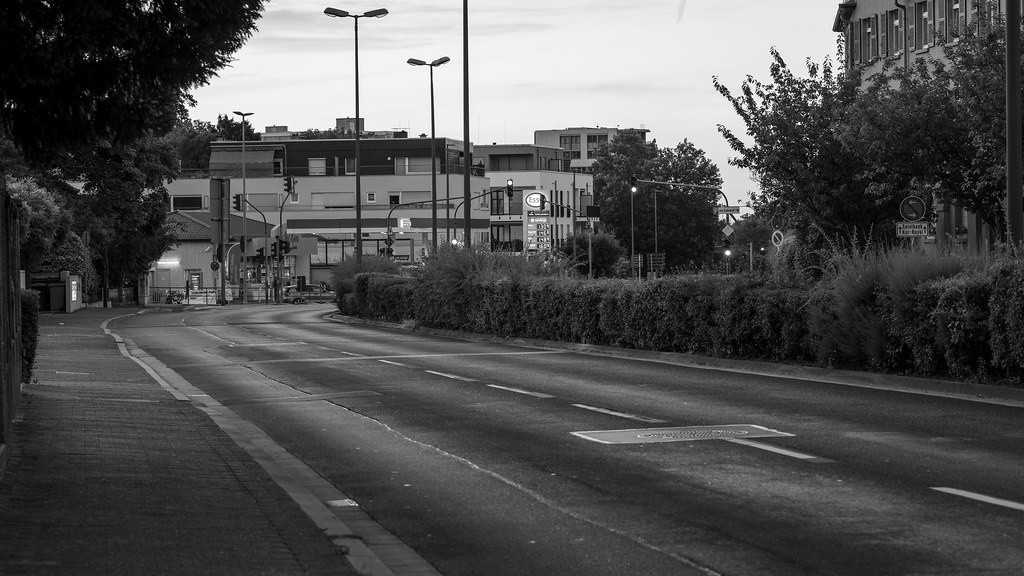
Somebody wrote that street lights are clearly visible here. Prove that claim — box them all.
[226,238,252,280]
[324,6,391,266]
[406,56,450,251]
[234,111,255,303]
[586,185,591,194]
[552,181,558,254]
[573,169,581,276]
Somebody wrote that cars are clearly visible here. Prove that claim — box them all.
[279,284,338,305]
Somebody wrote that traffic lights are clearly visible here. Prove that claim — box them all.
[271,242,276,259]
[283,176,294,196]
[540,194,545,210]
[256,247,264,264]
[233,194,242,212]
[631,176,637,193]
[281,241,290,254]
[725,240,730,258]
[507,180,515,199]
[760,245,767,255]
[452,239,458,249]
